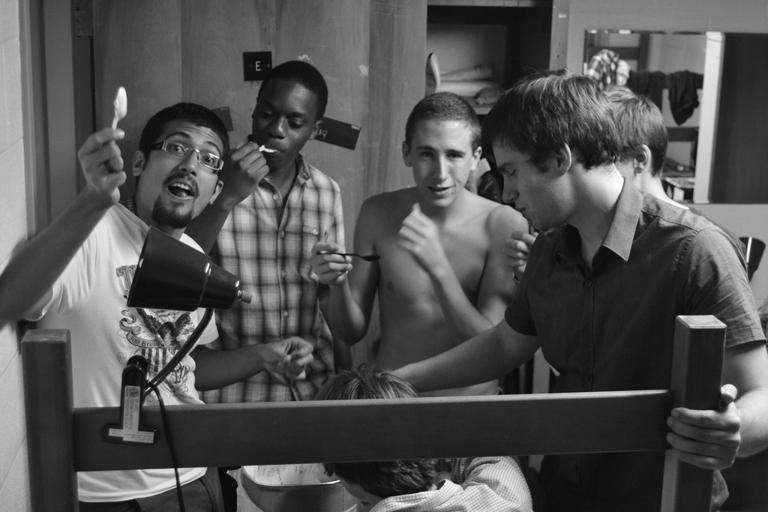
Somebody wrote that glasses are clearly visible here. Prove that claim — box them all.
[153,140,224,172]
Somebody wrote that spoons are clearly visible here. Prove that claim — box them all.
[335,253,381,261]
[109,86,128,144]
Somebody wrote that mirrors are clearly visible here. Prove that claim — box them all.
[578,27,725,208]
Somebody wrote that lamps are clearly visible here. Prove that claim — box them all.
[99,224,254,450]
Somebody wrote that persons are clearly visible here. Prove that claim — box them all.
[312,359,535,512]
[0,101,315,512]
[186,60,345,512]
[391,73,768,511]
[311,90,529,399]
[600,84,768,512]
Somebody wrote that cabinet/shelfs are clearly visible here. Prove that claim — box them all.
[93,0,573,370]
